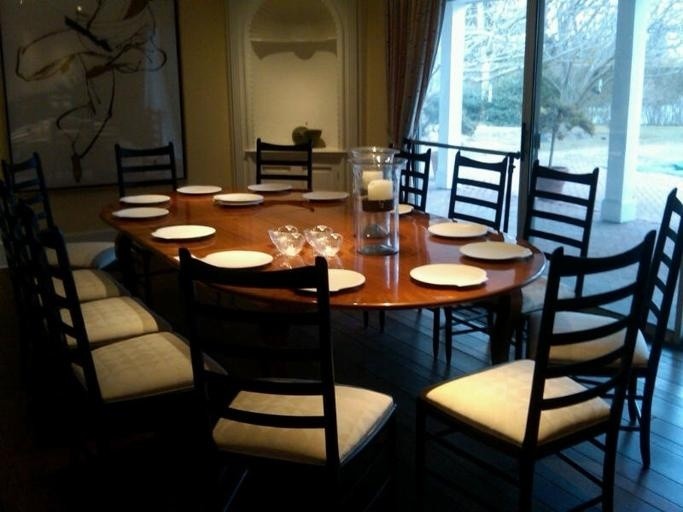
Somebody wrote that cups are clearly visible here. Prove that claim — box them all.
[345,146,400,257]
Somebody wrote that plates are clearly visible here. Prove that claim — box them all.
[297,268,366,294]
[459,241,535,261]
[429,221,487,239]
[392,204,414,216]
[120,184,350,270]
[408,264,489,289]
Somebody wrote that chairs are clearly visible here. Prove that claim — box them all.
[441,159,601,362]
[22,210,234,508]
[179,247,399,512]
[2,148,174,415]
[413,230,657,511]
[379,152,509,358]
[255,137,313,194]
[348,143,431,323]
[116,142,176,195]
[528,187,681,467]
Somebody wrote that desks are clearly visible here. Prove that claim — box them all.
[102,184,547,389]
